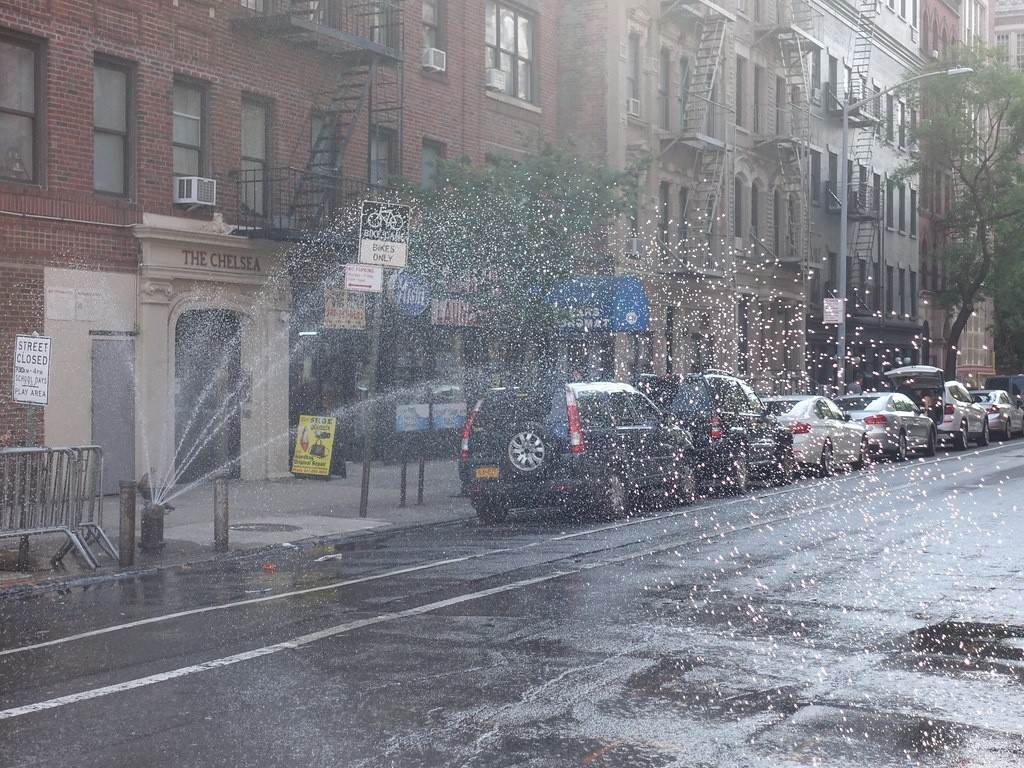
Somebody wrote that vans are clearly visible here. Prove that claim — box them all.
[984,374,1024,407]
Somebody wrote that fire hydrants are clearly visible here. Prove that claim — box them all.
[137,468,175,555]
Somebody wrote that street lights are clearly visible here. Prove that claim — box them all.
[835,65,974,395]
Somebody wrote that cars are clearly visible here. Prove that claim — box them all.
[835,392,939,460]
[883,364,988,451]
[758,395,870,477]
[970,389,1024,440]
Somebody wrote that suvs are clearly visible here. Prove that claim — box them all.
[458,381,699,523]
[635,369,793,495]
